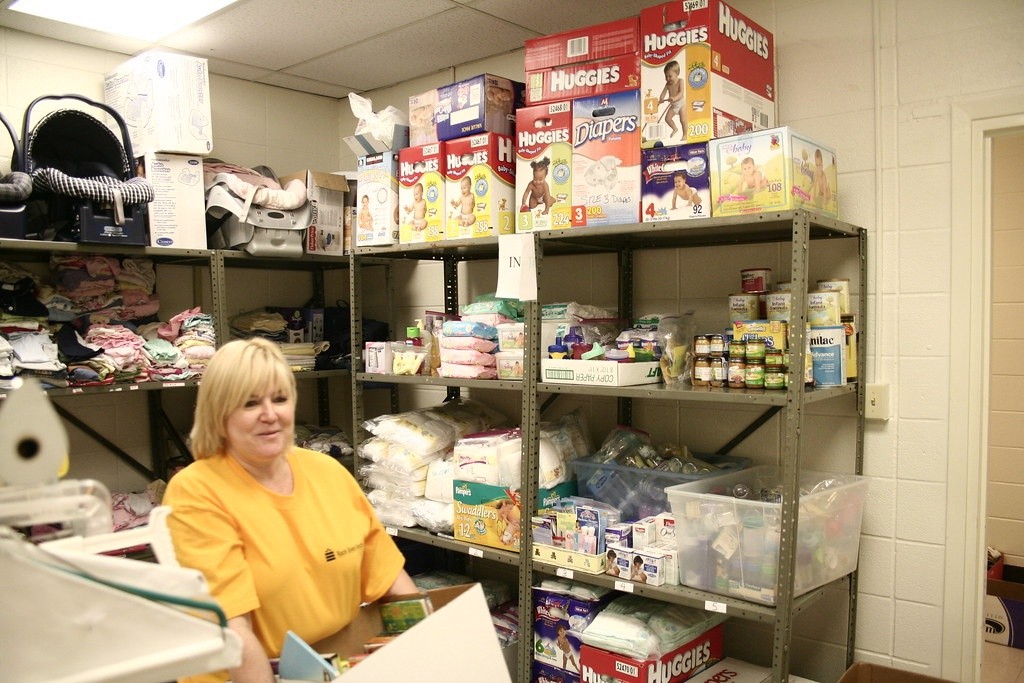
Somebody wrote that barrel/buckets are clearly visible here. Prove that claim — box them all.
[724,267,857,388]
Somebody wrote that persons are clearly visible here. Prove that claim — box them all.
[162,337,418,683]
[512,360,521,377]
[576,520,581,529]
[515,332,525,347]
[404,182,427,232]
[554,624,580,672]
[631,556,647,583]
[658,61,688,142]
[670,170,702,210]
[359,195,374,231]
[605,550,621,576]
[487,85,511,104]
[408,104,433,125]
[496,498,521,544]
[450,176,476,227]
[521,158,556,216]
[738,156,769,196]
[802,149,835,209]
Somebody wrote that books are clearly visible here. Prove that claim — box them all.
[268,590,435,683]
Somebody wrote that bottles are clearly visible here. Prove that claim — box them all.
[587,434,663,521]
[547,326,654,362]
[425,315,461,377]
[404,327,422,375]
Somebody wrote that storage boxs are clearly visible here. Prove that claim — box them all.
[398,141,446,244]
[835,661,954,683]
[266,307,326,342]
[494,322,567,354]
[324,581,513,683]
[640,142,712,223]
[344,180,357,247]
[102,47,213,161]
[540,358,663,387]
[639,0,777,148]
[572,89,642,226]
[731,320,787,351]
[987,554,1004,581]
[341,124,409,248]
[347,427,869,683]
[364,340,406,375]
[514,100,573,233]
[985,564,1024,650]
[277,169,350,256]
[708,126,840,220]
[69,205,148,245]
[445,131,516,239]
[495,352,550,380]
[408,72,526,149]
[143,153,209,250]
[524,14,641,104]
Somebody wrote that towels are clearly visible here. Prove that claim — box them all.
[0,255,216,388]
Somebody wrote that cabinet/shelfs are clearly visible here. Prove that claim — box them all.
[217,247,399,496]
[0,237,224,555]
[348,232,529,683]
[525,206,869,683]
